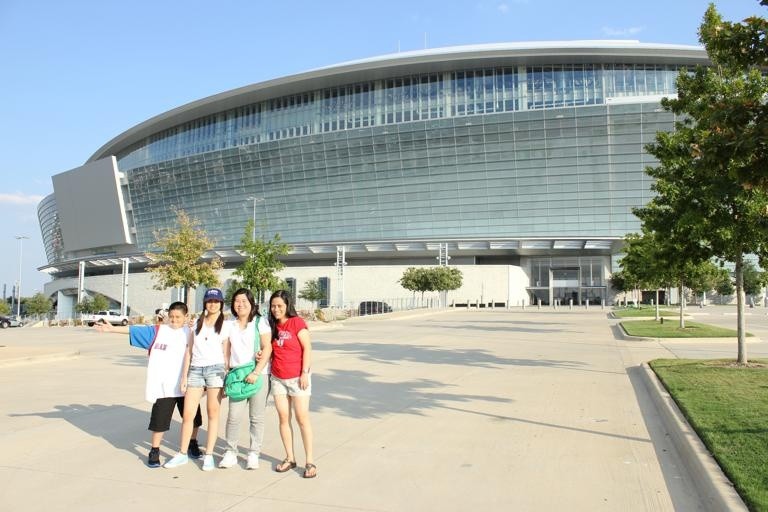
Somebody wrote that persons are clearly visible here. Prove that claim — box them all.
[217,288,273,468]
[255,290,317,478]
[93,300,202,467]
[162,288,229,471]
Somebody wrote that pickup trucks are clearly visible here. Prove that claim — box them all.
[82,310,130,327]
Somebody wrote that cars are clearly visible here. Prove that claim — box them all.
[0,316,10,329]
[7,318,23,328]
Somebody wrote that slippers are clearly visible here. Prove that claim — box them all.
[304,463,316,478]
[275,458,297,472]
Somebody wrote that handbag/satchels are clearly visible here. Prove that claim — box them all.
[224,362,262,401]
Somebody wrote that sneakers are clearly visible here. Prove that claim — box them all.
[188,440,203,458]
[163,455,188,468]
[218,455,237,468]
[202,456,214,471]
[148,451,160,467]
[246,456,258,469]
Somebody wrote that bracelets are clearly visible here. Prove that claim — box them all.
[302,368,310,374]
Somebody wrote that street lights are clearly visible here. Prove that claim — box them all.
[243,195,265,287]
[15,235,30,318]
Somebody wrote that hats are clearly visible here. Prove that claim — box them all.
[203,288,223,303]
